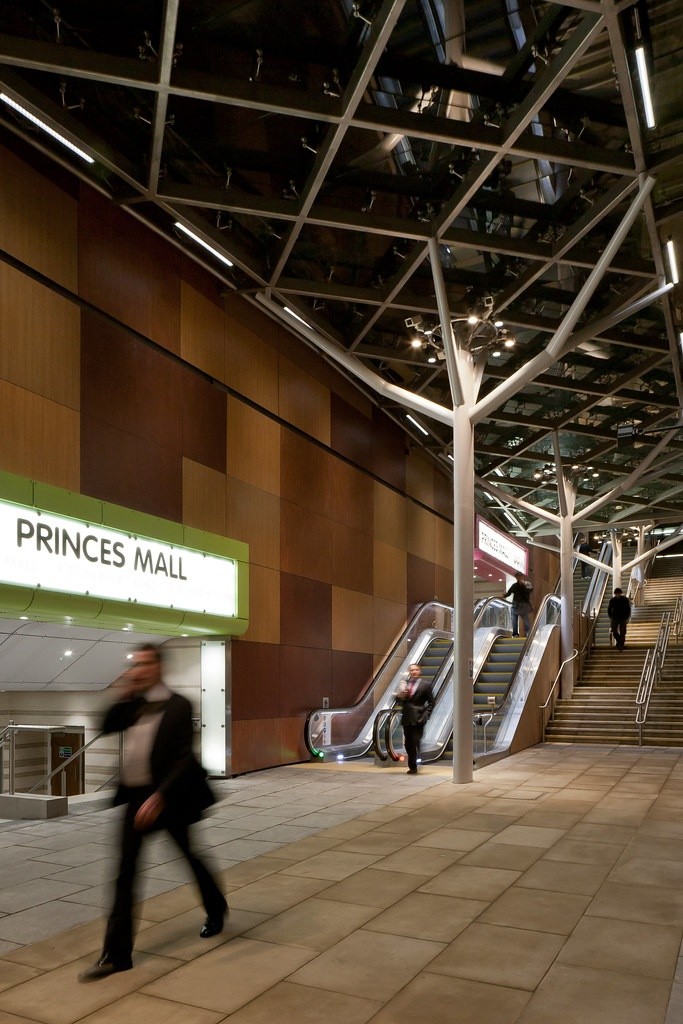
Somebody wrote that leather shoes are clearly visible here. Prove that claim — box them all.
[199,900,227,937]
[78,951,133,981]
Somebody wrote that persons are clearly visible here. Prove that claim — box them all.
[393,664,435,774]
[76,644,230,984]
[577,538,601,579]
[607,588,631,650]
[499,571,534,638]
